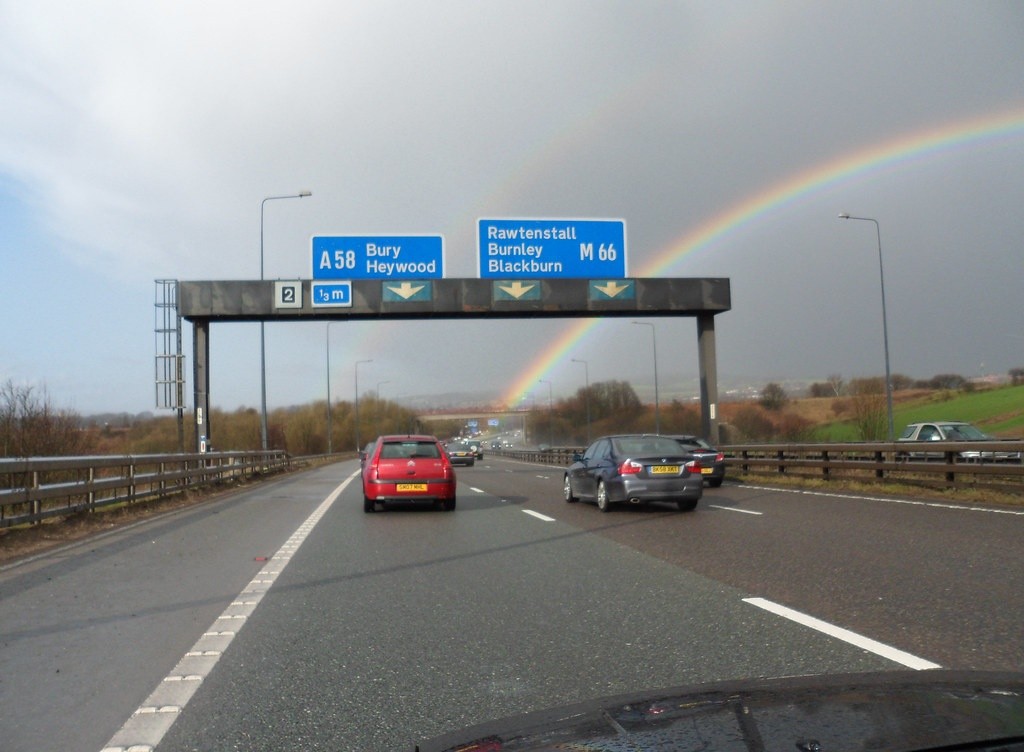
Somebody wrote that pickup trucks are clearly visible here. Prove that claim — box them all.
[466,440,484,460]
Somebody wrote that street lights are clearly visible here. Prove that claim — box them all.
[571,357,593,441]
[839,211,896,442]
[257,189,314,445]
[355,358,373,451]
[633,320,662,433]
[375,380,388,400]
[537,379,554,446]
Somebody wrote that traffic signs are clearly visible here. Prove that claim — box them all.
[314,234,443,279]
[478,219,626,277]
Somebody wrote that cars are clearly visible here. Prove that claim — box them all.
[447,444,474,466]
[362,434,456,513]
[438,429,521,449]
[563,433,703,512]
[359,441,378,473]
[896,420,1021,464]
[658,432,726,488]
